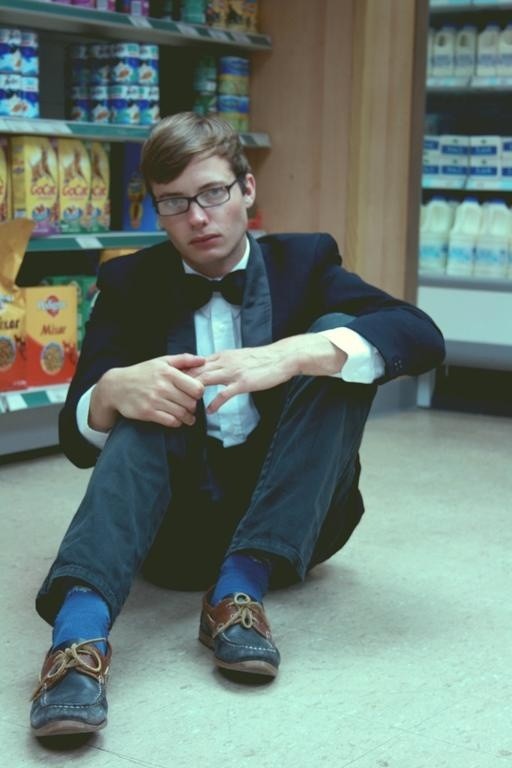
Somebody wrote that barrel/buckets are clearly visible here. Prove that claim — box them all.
[417,20,512,292]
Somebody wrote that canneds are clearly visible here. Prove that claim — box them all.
[0,25,251,133]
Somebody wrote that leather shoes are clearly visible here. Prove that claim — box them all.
[197,582,281,677]
[29,636,110,739]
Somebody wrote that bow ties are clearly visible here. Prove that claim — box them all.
[175,269,246,313]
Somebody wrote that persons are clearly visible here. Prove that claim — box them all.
[26,109,448,742]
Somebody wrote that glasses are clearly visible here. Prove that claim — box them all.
[152,178,239,217]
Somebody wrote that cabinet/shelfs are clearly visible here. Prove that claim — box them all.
[1,1,270,469]
[398,3,512,408]
